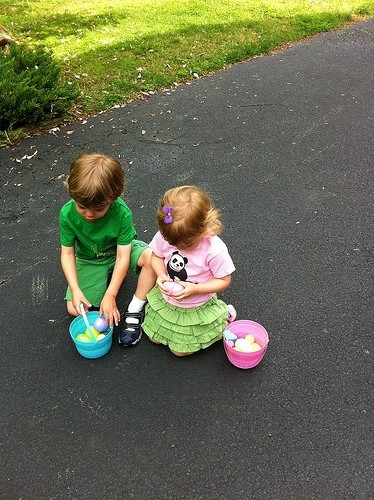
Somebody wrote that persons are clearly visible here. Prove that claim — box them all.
[59,152,157,345]
[142,186,236,356]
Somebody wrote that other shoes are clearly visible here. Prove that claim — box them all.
[227,304,237,323]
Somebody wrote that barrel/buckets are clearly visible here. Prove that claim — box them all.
[222,320,269,369]
[68,303,114,359]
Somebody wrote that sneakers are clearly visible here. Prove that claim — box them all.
[118,307,144,347]
